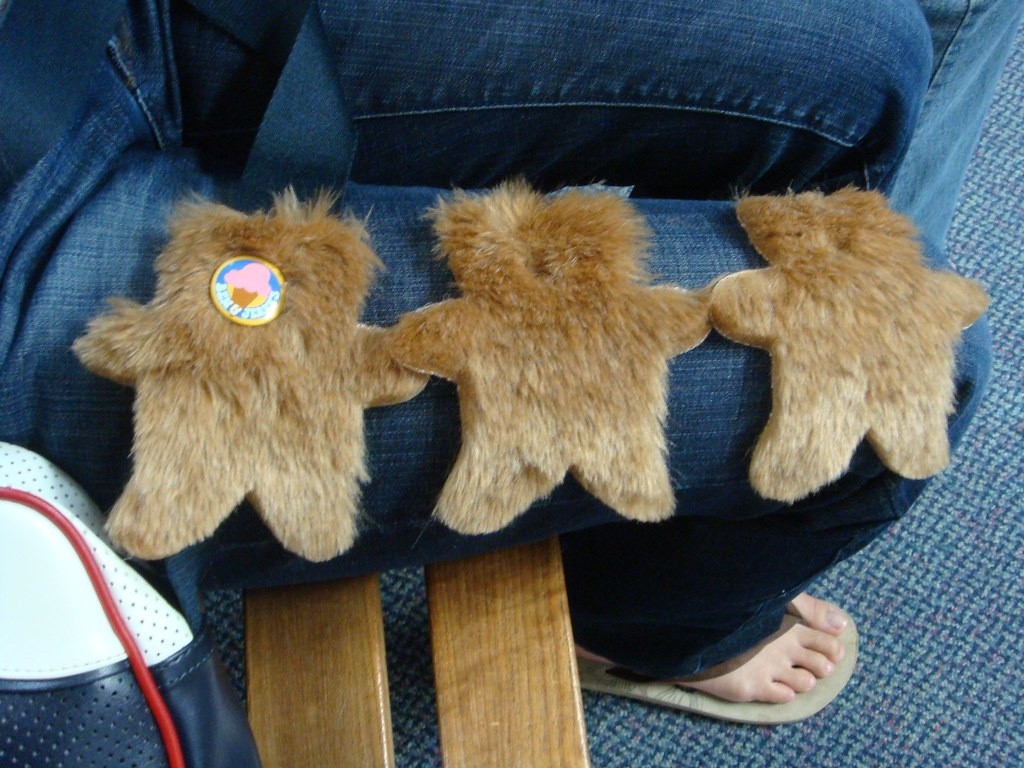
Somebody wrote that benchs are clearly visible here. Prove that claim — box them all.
[200,529,600,767]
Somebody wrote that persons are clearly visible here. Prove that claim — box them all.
[0,0,994,724]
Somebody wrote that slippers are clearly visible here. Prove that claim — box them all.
[575,587,859,724]
